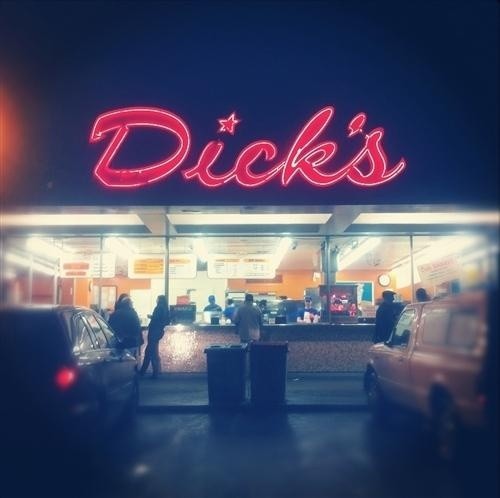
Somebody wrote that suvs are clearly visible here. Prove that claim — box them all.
[361,292,498,455]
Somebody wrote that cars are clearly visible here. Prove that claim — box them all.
[2,302,140,445]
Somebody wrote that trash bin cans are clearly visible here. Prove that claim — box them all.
[249,340,288,434]
[204,344,247,434]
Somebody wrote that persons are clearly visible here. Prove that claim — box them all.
[203,295,222,325]
[106,287,170,378]
[296,296,319,323]
[416,288,427,303]
[232,294,263,343]
[373,290,396,343]
[223,299,236,321]
[330,299,344,313]
